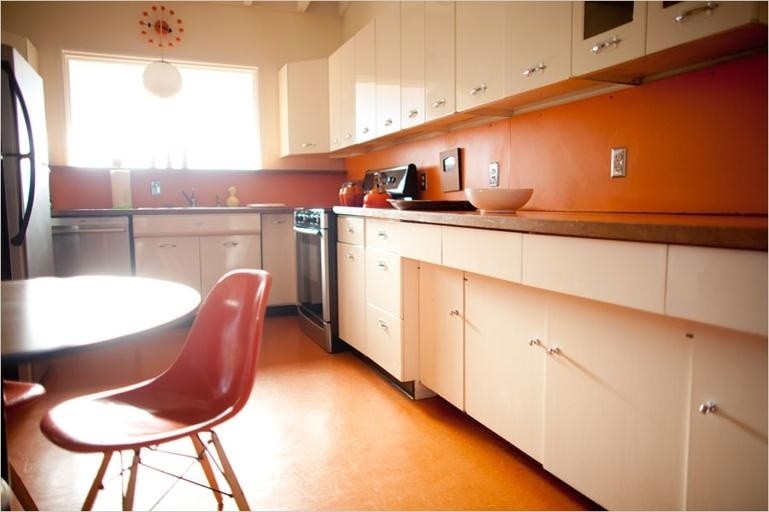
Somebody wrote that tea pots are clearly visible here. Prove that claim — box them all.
[363,182,393,208]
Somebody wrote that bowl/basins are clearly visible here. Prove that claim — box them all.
[464,187,534,214]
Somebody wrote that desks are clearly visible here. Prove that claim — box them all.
[0,274,201,361]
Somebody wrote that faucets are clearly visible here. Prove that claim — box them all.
[177,187,200,206]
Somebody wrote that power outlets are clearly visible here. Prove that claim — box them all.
[420,173,427,192]
[488,163,498,186]
[611,147,626,179]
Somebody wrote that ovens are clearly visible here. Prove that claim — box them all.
[294,209,337,354]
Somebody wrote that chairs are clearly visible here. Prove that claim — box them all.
[38,268,271,511]
[1,379,46,512]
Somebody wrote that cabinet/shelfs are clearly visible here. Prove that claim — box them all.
[262,215,297,307]
[400,222,768,510]
[337,216,366,357]
[131,213,262,300]
[454,1,639,116]
[398,0,512,133]
[366,220,436,400]
[355,1,445,146]
[278,58,329,159]
[329,35,389,157]
[572,1,768,85]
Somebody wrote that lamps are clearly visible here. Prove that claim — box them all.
[141,1,183,98]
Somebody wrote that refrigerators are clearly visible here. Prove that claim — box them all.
[0,45,56,389]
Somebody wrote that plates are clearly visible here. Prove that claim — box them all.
[383,198,479,211]
